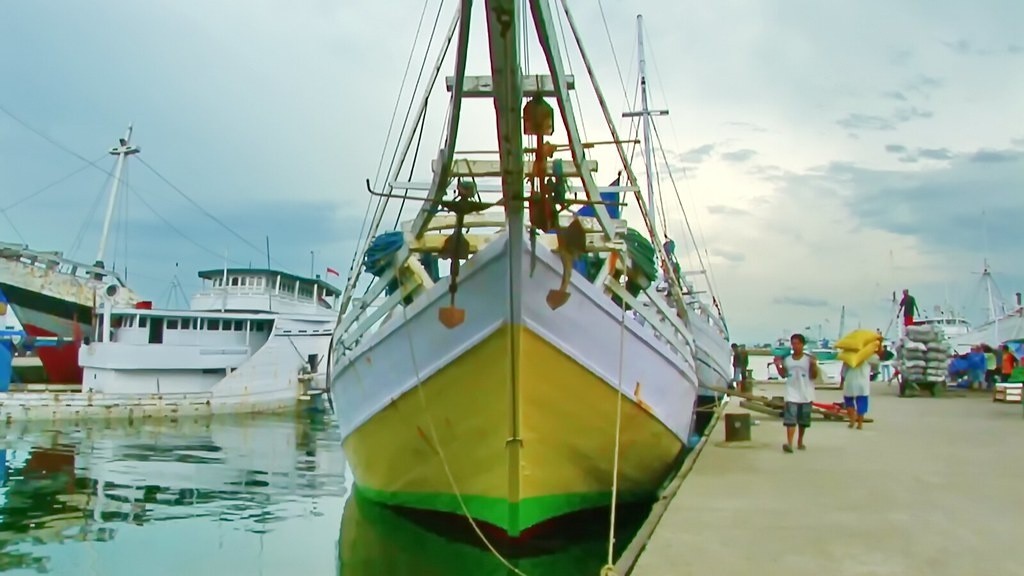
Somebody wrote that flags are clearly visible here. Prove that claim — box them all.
[327,268,339,276]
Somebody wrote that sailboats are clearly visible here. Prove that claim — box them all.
[326,0,737,542]
[0,122,269,387]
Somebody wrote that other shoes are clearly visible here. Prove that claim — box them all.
[848,422,854,428]
[857,425,862,429]
[798,445,806,450]
[783,445,793,453]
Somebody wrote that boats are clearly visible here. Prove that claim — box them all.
[765,257,1024,381]
[75,232,341,412]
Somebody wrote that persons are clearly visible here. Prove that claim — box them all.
[897,289,920,325]
[732,343,748,385]
[840,337,881,430]
[880,345,894,382]
[951,343,1019,390]
[773,333,817,452]
